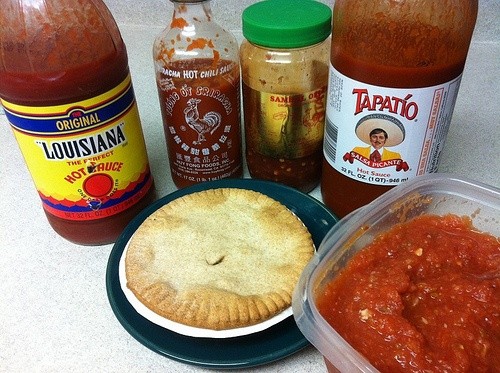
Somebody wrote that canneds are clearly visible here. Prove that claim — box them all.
[239,0,333,194]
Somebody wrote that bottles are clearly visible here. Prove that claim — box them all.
[320,0,479,232]
[152,0,243,189]
[0,0,155,246]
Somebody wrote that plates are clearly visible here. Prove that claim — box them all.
[105,178,347,370]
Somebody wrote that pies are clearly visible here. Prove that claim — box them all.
[125,189,316,330]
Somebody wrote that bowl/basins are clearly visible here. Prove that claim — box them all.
[293,172,500,373]
